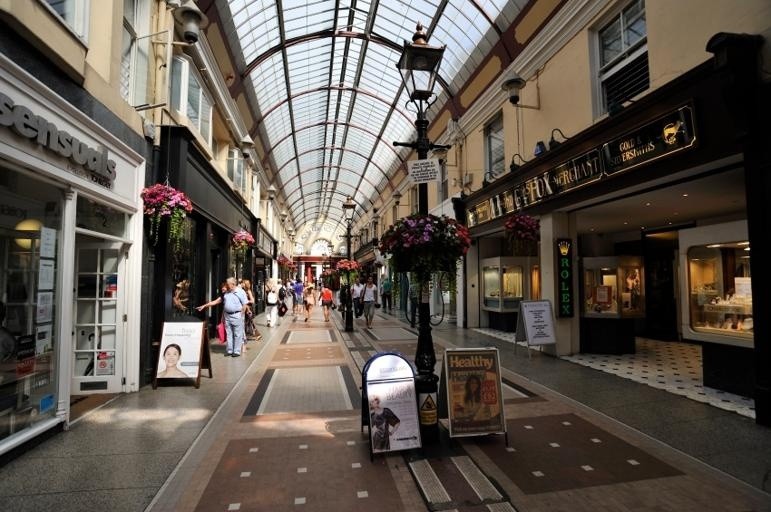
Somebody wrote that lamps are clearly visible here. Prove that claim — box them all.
[259,184,278,201]
[276,209,296,239]
[226,133,255,160]
[482,171,499,188]
[510,154,529,172]
[606,86,636,117]
[151,0,209,46]
[550,129,573,151]
[461,186,476,200]
[392,189,409,207]
[501,68,541,111]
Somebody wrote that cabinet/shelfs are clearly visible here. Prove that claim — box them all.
[0,229,59,470]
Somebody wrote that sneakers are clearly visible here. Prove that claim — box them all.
[224,348,240,358]
[244,329,263,342]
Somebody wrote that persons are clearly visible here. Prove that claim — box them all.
[262,276,378,329]
[457,375,496,422]
[174,274,192,314]
[195,281,229,323]
[383,276,391,296]
[158,342,191,378]
[380,272,393,314]
[221,277,250,357]
[368,394,403,454]
[241,278,262,341]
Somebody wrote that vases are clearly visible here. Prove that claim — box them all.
[391,243,456,272]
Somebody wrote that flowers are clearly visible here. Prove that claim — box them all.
[336,259,359,287]
[503,215,539,250]
[232,228,255,260]
[377,212,471,307]
[142,183,194,252]
[322,269,337,284]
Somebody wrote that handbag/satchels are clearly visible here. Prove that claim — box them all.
[278,302,289,317]
[267,292,278,305]
[356,302,365,317]
[216,320,227,344]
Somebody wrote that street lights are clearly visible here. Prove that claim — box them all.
[326,240,334,269]
[339,195,360,331]
[393,19,451,446]
